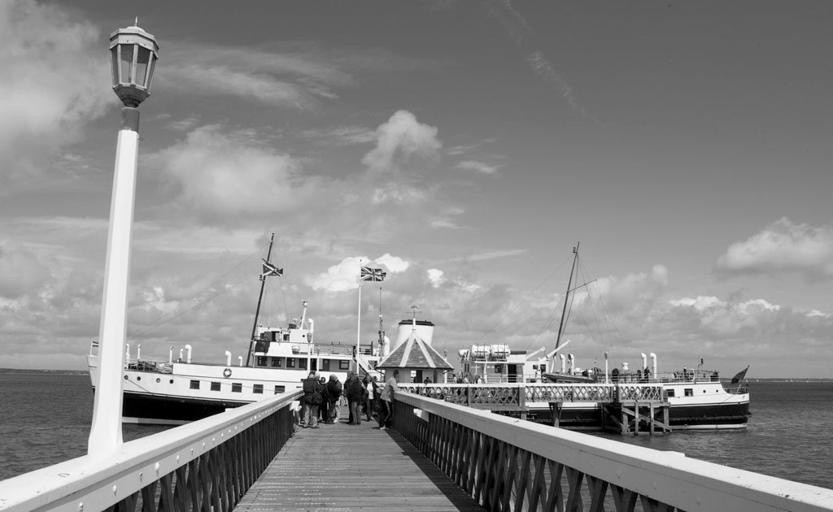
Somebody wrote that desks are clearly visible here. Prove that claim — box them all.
[82,11,162,458]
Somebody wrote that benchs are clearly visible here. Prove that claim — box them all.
[360,265,384,282]
[262,258,283,277]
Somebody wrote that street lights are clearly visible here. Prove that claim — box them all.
[311,393,322,404]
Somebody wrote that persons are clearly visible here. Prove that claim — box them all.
[301,369,487,431]
[643,365,652,384]
